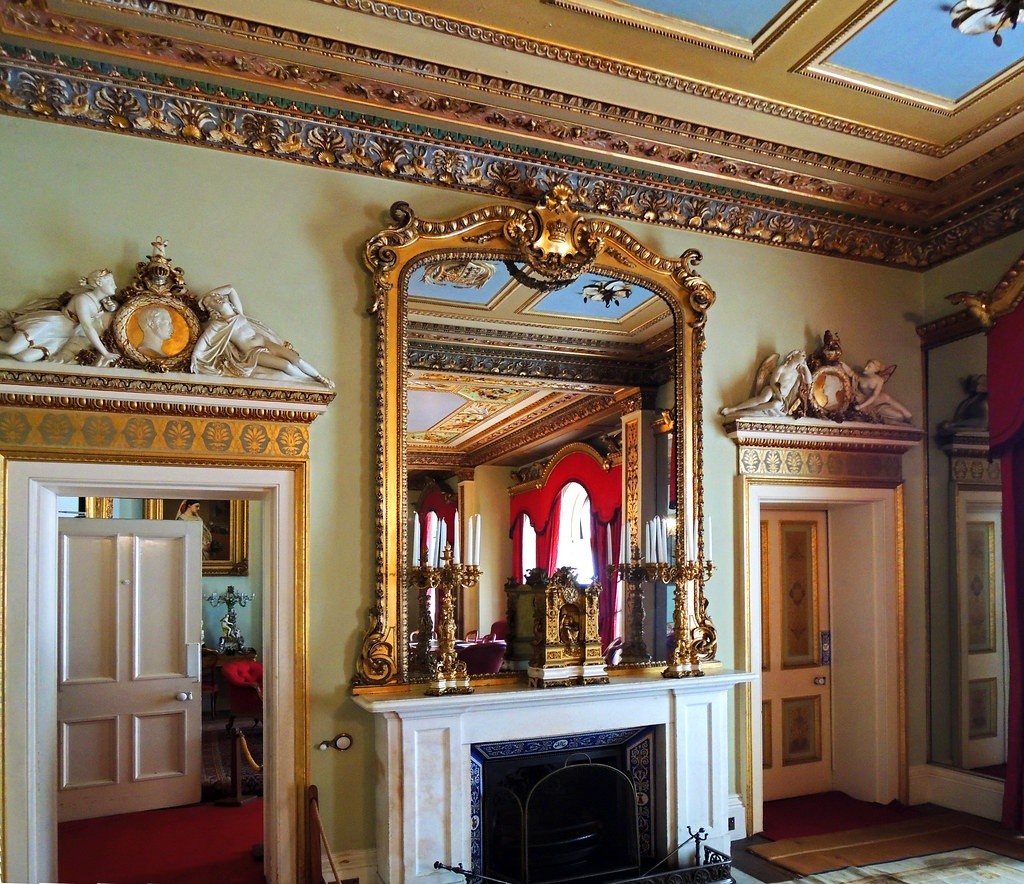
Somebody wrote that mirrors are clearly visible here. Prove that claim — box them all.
[345,180,722,700]
[915,309,1011,786]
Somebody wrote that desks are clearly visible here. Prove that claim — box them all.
[203,648,258,668]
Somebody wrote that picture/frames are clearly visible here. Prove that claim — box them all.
[143,498,250,577]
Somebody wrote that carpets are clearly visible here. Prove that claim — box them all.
[202,719,263,799]
[748,807,1024,876]
[757,790,925,843]
[766,844,1024,884]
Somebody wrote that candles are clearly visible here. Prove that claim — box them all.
[651,516,657,564]
[694,517,699,560]
[708,514,713,560]
[429,510,437,566]
[645,515,667,563]
[453,507,460,565]
[412,509,418,567]
[472,512,481,565]
[686,515,694,560]
[433,517,448,569]
[656,515,664,563]
[467,515,473,566]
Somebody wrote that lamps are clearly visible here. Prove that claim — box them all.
[949,0,1024,47]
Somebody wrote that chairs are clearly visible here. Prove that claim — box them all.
[223,660,263,731]
[202,651,220,720]
